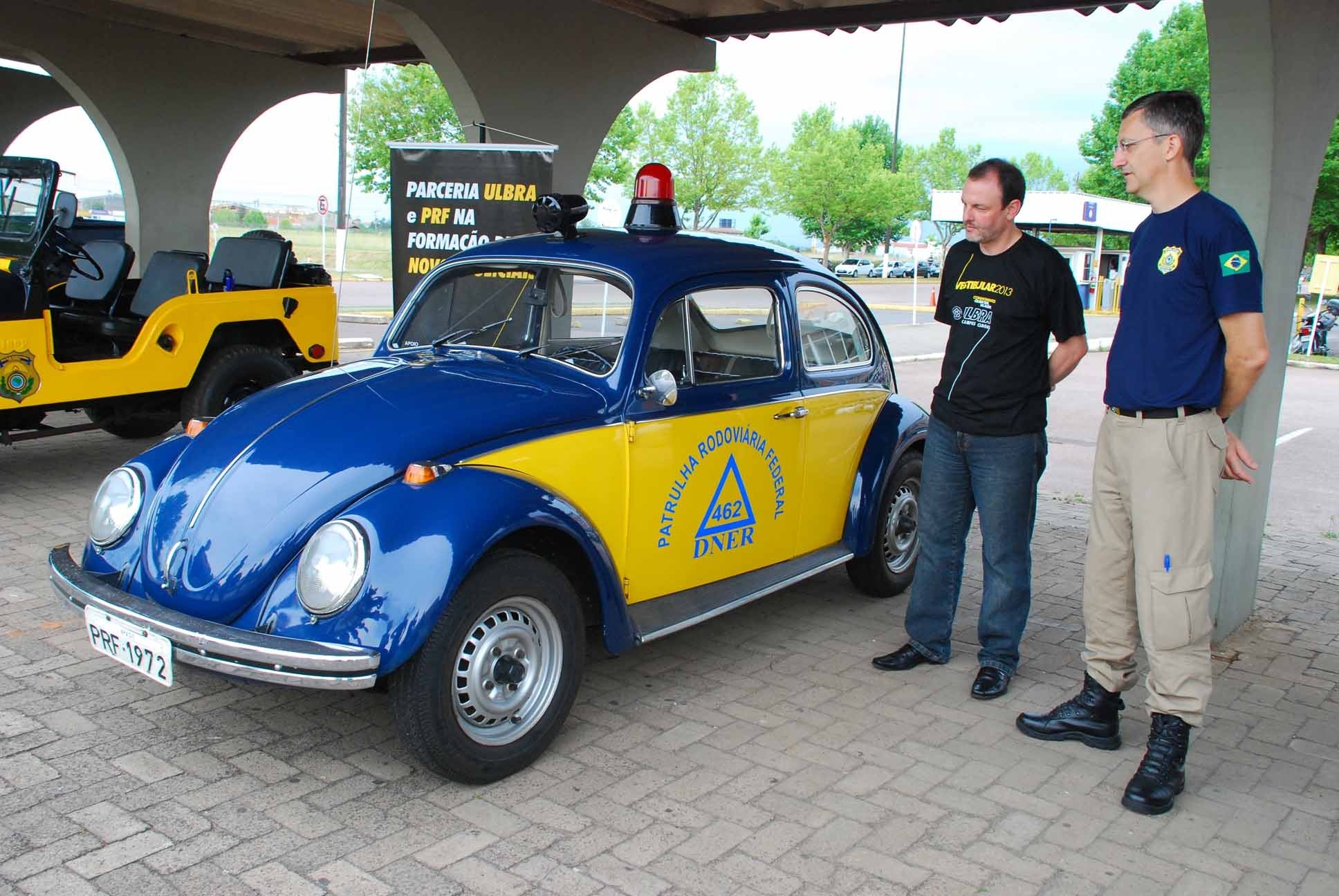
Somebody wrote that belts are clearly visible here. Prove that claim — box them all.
[1111,400,1208,419]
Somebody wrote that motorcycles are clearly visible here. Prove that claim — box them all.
[1288,298,1339,355]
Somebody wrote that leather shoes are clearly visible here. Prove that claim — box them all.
[971,665,1010,698]
[872,644,942,671]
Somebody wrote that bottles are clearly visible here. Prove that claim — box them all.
[222,269,233,292]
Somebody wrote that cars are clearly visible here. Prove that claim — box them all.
[0,151,341,439]
[807,255,941,280]
[48,162,937,789]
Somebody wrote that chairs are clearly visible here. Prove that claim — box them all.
[61,249,210,357]
[205,237,289,291]
[51,238,136,348]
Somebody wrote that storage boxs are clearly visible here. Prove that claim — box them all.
[1327,299,1339,315]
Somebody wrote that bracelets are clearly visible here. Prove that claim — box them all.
[1221,417,1229,424]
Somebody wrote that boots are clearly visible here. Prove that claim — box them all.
[1017,671,1126,750]
[1122,713,1191,815]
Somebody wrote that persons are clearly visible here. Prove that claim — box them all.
[1111,259,1118,271]
[1016,88,1270,814]
[872,158,1089,698]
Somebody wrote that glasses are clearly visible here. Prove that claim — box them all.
[1113,132,1171,157]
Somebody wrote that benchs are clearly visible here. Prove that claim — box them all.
[597,342,778,383]
